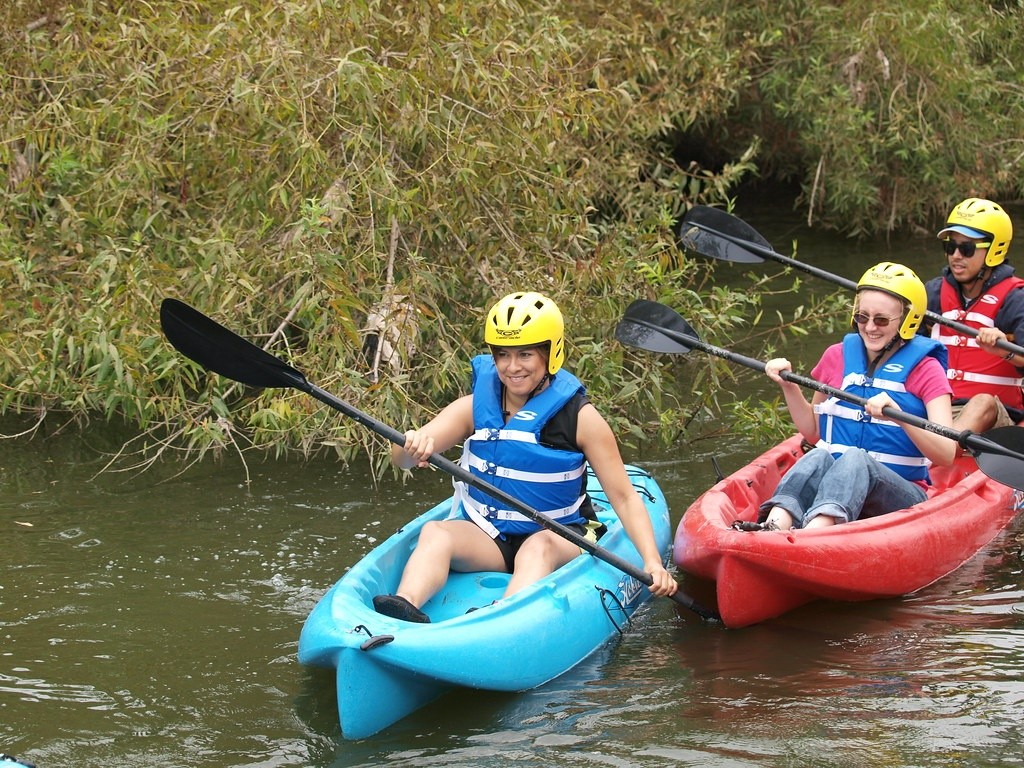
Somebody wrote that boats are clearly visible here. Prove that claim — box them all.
[677,431,1023,628]
[297,461,674,745]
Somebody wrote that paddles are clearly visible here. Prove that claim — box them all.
[159,295,723,621]
[617,297,1024,461]
[682,204,1024,355]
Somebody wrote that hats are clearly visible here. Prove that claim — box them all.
[937,225,988,239]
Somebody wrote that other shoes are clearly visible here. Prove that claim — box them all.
[373,593,430,623]
[731,519,781,531]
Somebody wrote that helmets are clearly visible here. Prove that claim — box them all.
[850,262,927,339]
[484,292,564,375]
[943,197,1013,267]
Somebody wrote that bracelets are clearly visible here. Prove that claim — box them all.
[1004,352,1015,360]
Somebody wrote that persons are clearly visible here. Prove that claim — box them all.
[925,198,1024,458]
[731,261,954,532]
[372,292,677,624]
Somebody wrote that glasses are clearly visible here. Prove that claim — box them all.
[942,238,991,257]
[851,309,899,326]
